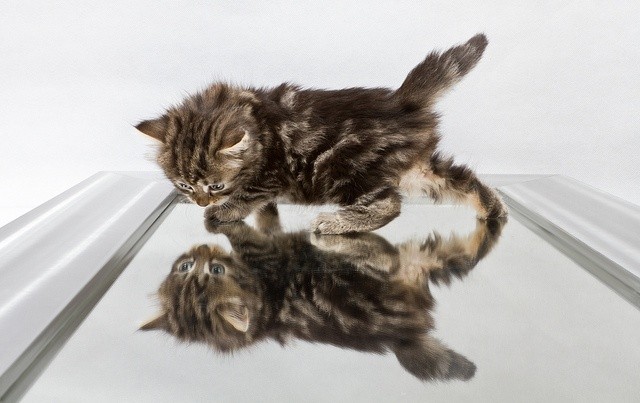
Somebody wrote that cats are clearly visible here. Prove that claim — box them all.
[134,220,508,384]
[133,31,510,235]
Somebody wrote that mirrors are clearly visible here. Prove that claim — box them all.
[0,169,640,401]
